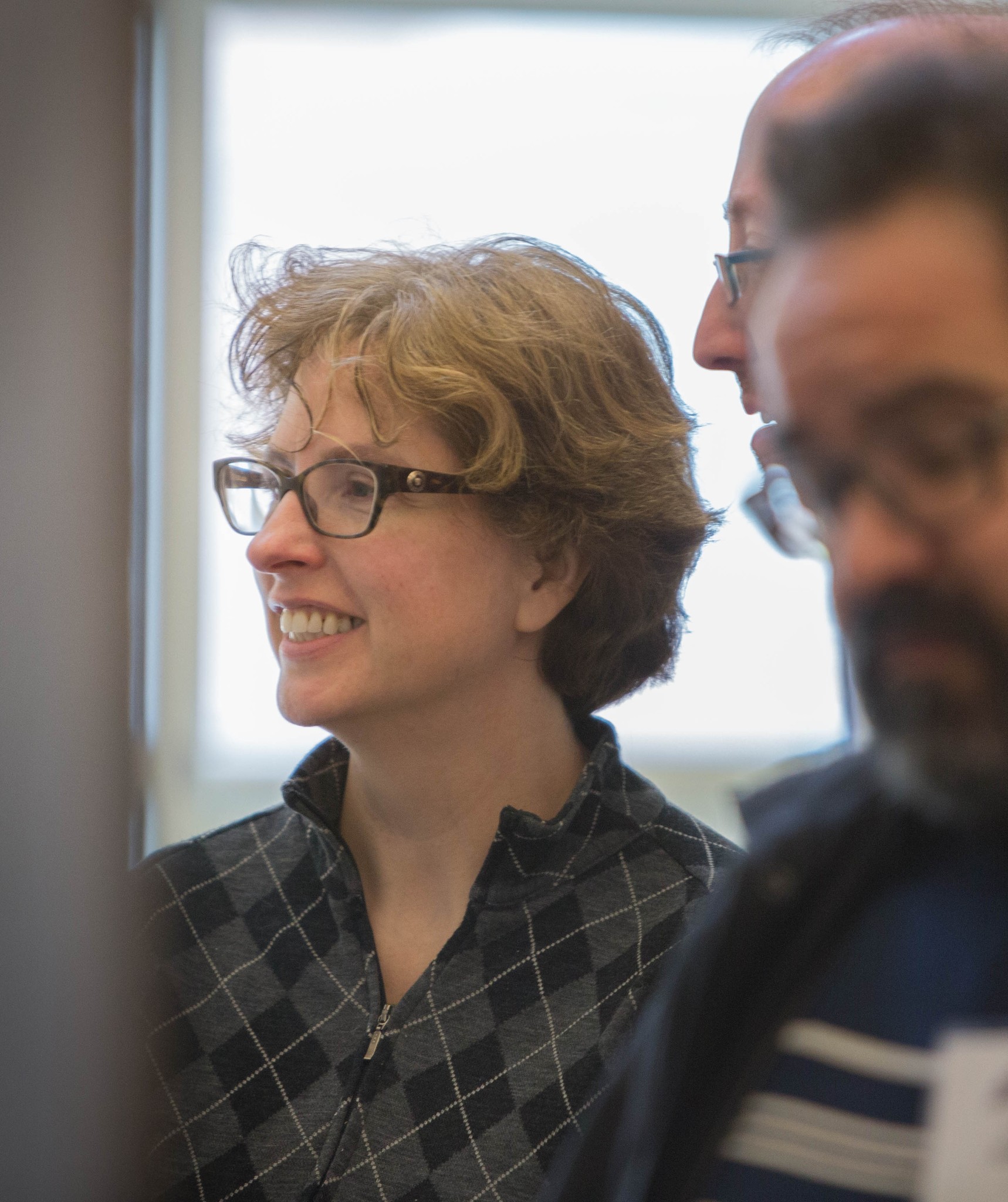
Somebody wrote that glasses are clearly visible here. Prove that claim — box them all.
[216,458,514,537]
[713,246,780,305]
[738,398,988,561]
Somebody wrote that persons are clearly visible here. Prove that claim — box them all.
[536,0,1008,1202]
[132,236,751,1202]
